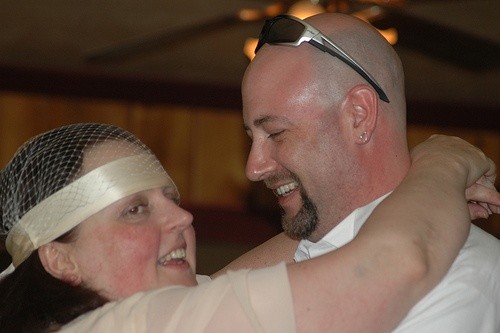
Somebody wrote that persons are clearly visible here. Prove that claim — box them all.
[0,122,500,333]
[240,14,500,333]
